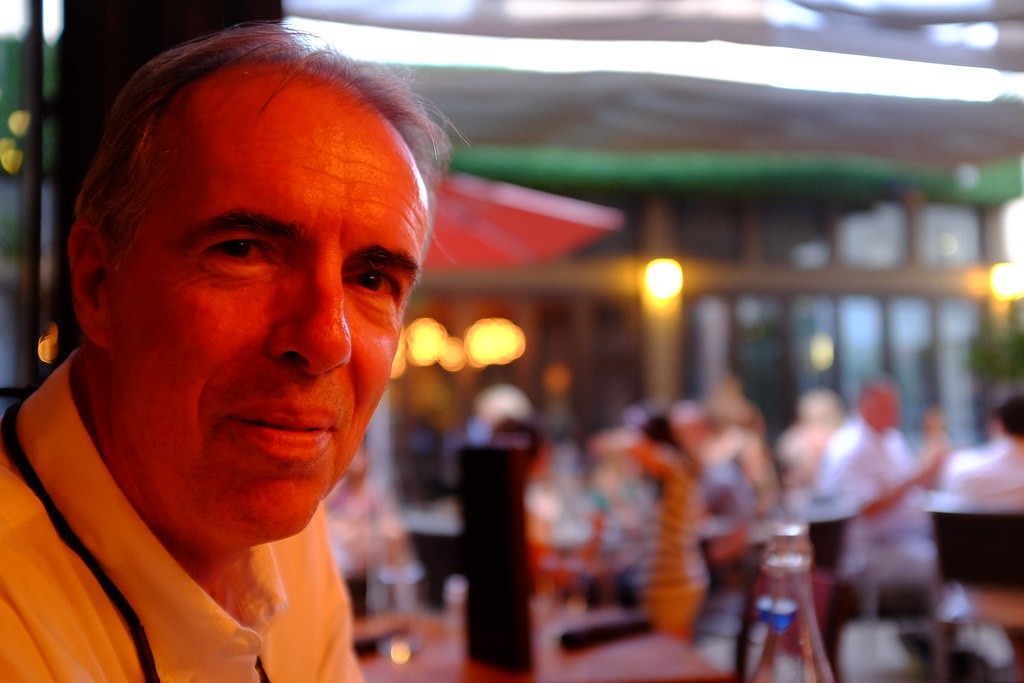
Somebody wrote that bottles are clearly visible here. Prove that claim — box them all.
[740,525,836,683]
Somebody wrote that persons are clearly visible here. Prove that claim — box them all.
[0,27,454,683]
[315,366,1024,682]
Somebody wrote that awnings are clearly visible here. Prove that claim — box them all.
[428,165,616,270]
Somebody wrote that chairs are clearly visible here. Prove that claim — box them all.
[808,512,1024,683]
